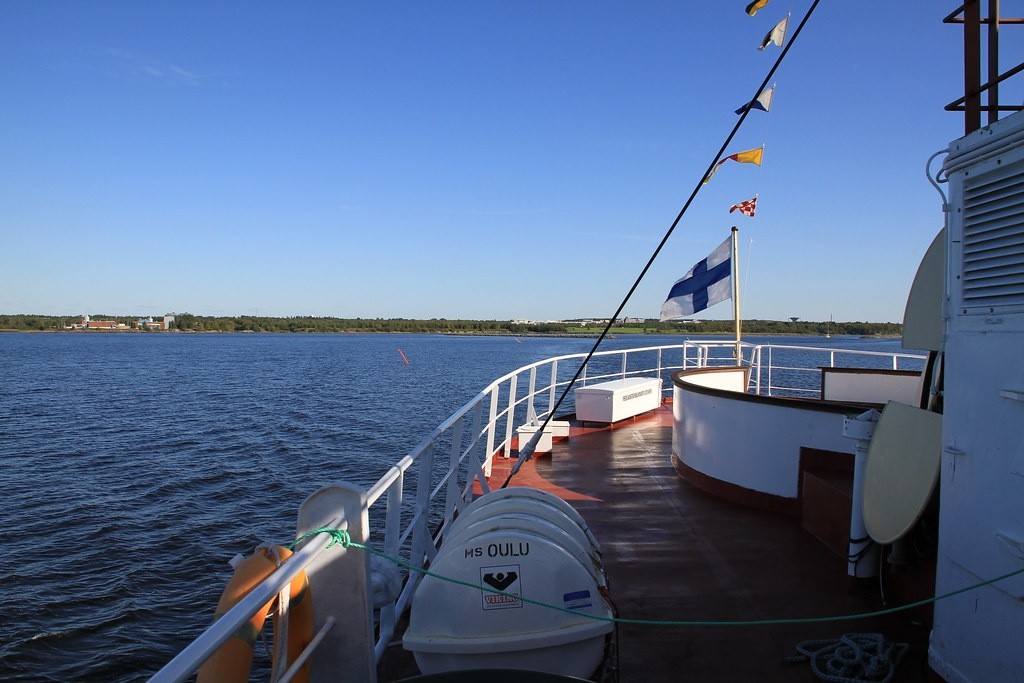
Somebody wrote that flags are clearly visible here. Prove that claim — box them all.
[659,234,735,322]
[729,197,758,217]
[703,147,763,184]
[757,17,788,51]
[734,88,773,115]
[745,0,768,16]
